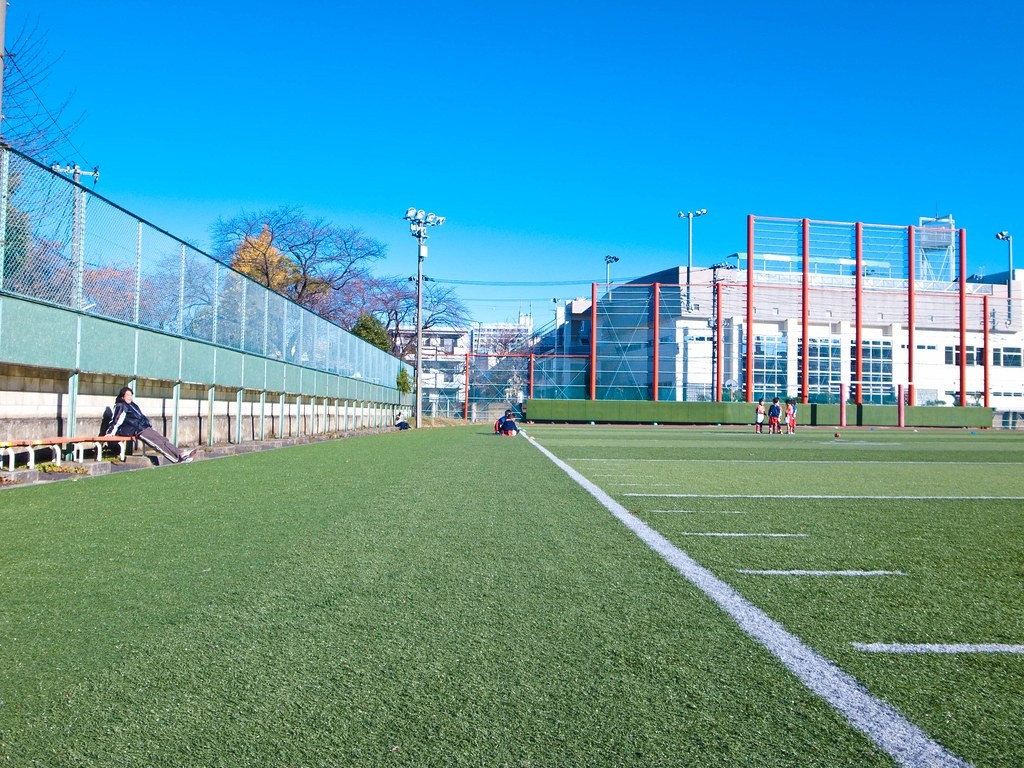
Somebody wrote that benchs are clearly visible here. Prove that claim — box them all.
[1,436,135,470]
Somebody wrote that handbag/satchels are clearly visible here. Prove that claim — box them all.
[118,426,139,450]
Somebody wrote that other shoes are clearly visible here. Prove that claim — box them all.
[757,430,796,434]
[180,458,194,464]
[181,449,196,459]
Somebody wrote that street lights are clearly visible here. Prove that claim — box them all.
[678,208,708,312]
[995,231,1013,324]
[403,207,446,429]
[604,254,619,293]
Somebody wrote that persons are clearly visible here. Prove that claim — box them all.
[755,399,768,434]
[768,397,798,434]
[395,412,411,431]
[104,386,198,463]
[498,409,520,436]
